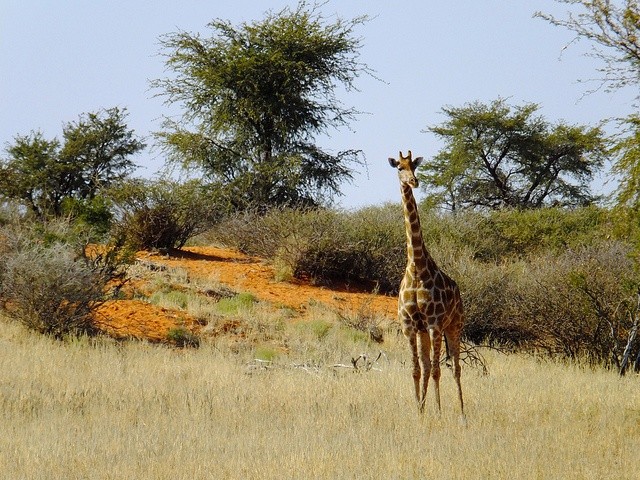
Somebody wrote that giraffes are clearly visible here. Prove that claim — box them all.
[388,152,467,426]
